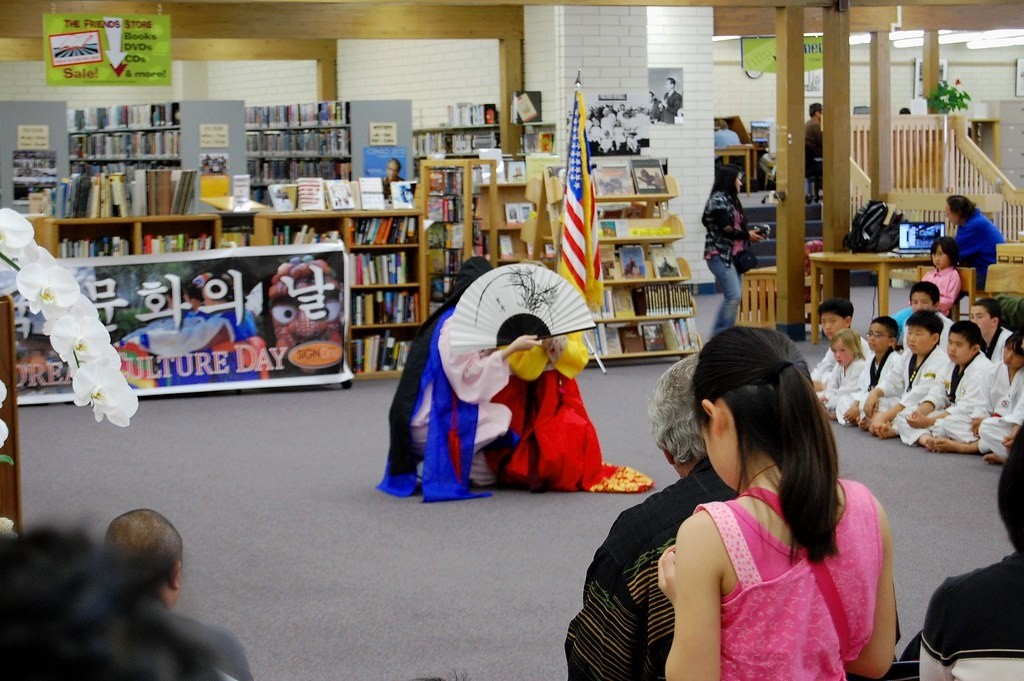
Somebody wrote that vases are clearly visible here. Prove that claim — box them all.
[936,105,951,114]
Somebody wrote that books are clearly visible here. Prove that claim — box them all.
[52,101,554,374]
[582,158,699,354]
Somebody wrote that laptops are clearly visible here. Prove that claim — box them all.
[897,221,945,254]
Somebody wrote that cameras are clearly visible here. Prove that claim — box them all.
[753,225,766,235]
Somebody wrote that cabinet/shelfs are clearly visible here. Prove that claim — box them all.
[256,210,426,381]
[481,161,558,281]
[68,100,245,210]
[419,158,524,316]
[590,154,703,360]
[24,215,222,262]
[413,124,558,315]
[246,100,412,206]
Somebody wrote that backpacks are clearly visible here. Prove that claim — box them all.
[850,199,904,253]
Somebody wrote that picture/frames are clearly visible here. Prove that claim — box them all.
[630,159,669,195]
[519,203,533,222]
[505,204,519,223]
[591,163,635,196]
[914,57,948,100]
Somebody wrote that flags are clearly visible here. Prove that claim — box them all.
[543,89,605,340]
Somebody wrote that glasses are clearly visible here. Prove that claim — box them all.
[866,331,889,339]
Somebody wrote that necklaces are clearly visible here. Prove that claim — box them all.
[749,463,775,486]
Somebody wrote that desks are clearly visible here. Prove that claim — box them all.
[716,148,751,198]
[808,252,928,346]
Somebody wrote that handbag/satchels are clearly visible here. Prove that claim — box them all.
[733,250,759,274]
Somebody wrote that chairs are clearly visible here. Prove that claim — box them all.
[919,265,976,324]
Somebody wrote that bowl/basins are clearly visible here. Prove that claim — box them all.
[287,339,343,375]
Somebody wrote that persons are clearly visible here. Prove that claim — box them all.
[624,254,637,278]
[895,423,1024,681]
[587,78,681,156]
[805,102,823,196]
[701,164,763,335]
[714,119,739,146]
[376,256,541,503]
[563,353,742,681]
[810,281,1024,467]
[892,236,961,340]
[383,158,405,199]
[0,508,253,681]
[946,194,1006,290]
[656,330,895,681]
[491,330,655,494]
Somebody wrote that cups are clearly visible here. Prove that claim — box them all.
[910,99,927,115]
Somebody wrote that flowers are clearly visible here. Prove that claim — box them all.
[0,208,139,536]
[927,77,971,110]
